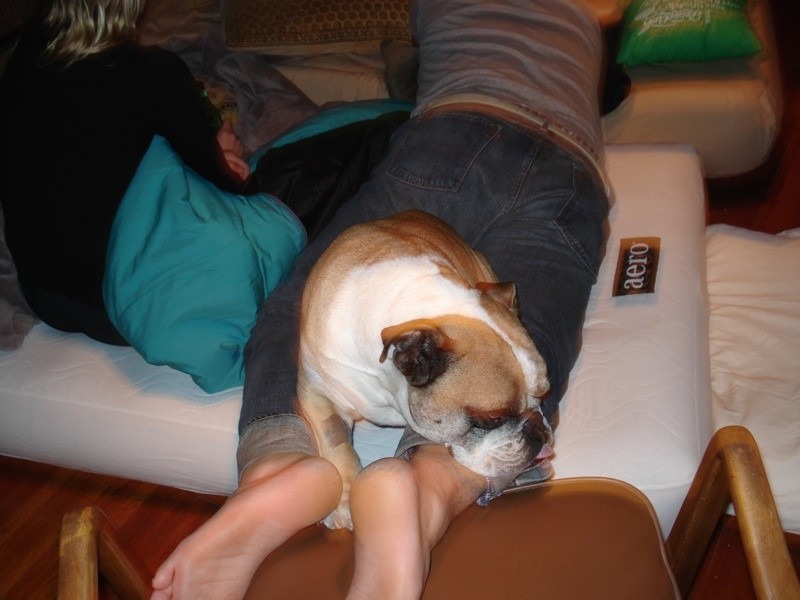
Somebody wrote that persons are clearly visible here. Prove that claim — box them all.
[0,0,416,395]
[151,0,632,600]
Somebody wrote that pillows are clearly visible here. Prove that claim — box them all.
[617,0,764,66]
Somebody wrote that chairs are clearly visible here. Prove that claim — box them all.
[58,427,800,600]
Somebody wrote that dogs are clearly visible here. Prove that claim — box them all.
[296,211,552,532]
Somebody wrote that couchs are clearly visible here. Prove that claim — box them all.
[136,0,784,177]
